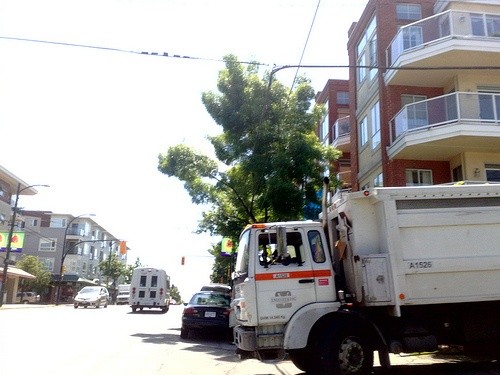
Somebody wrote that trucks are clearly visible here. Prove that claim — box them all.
[117,283,131,304]
[230,177,500,375]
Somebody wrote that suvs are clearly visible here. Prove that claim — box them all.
[16,292,41,304]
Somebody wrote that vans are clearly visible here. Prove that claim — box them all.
[130,267,170,313]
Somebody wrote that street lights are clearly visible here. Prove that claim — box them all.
[57,213,119,305]
[0,183,51,306]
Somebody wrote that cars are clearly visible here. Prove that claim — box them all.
[74,286,110,308]
[181,284,231,338]
